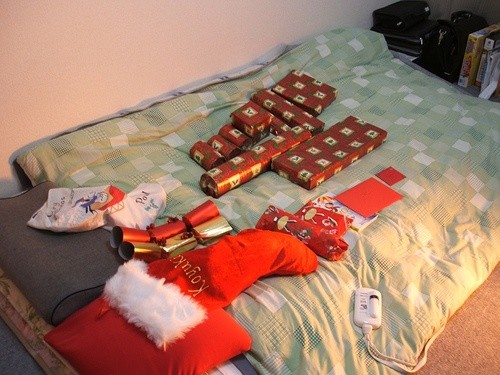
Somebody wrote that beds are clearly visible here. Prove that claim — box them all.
[0,24,498,375]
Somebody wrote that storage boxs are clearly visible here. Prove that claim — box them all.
[233,102,272,145]
[273,68,338,117]
[271,114,388,190]
[253,88,326,137]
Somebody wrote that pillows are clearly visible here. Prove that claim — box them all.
[0,180,127,328]
[44,294,251,375]
